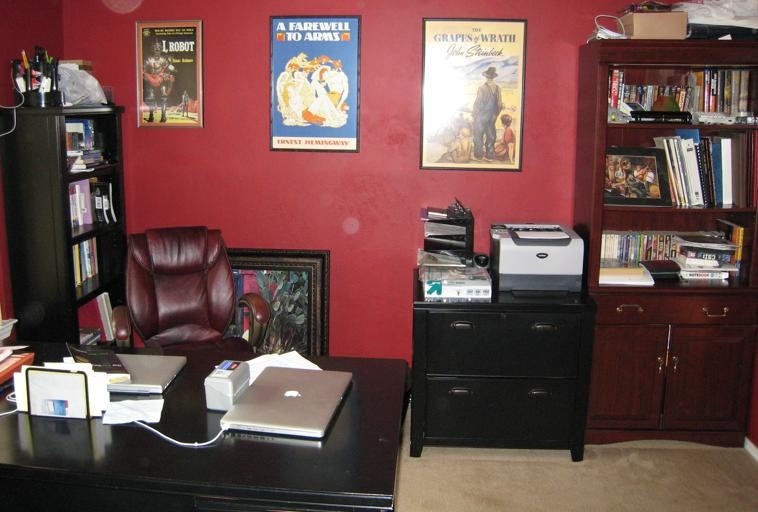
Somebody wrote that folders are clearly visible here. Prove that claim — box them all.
[68,178,94,225]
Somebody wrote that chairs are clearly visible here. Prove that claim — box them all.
[112,226,270,352]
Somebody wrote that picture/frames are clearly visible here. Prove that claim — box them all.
[269,15,362,153]
[419,17,528,172]
[226,248,330,355]
[135,19,203,129]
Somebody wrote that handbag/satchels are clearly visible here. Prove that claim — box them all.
[585,13,628,46]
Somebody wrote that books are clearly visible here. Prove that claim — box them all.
[598,68,758,287]
[65,120,116,348]
[61,343,131,382]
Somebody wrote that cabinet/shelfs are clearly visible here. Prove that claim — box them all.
[574,40,758,448]
[0,106,126,345]
[410,268,597,462]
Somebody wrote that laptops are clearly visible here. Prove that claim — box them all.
[219,365,354,441]
[107,352,187,394]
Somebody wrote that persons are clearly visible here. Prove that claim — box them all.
[440,67,516,163]
[143,38,190,123]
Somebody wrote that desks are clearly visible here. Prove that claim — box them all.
[0,339,410,512]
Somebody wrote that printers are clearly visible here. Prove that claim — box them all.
[489,221,585,296]
